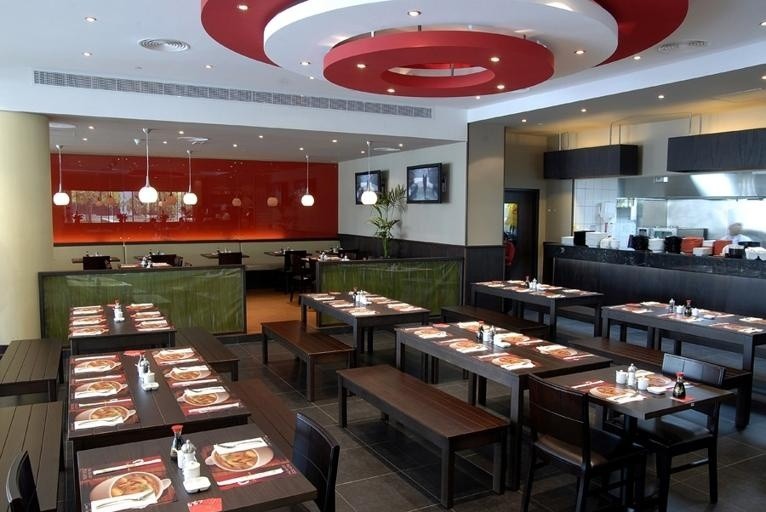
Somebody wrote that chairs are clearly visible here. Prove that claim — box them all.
[6,450,40,512]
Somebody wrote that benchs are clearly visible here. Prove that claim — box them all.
[0,338,64,402]
[0,400,63,512]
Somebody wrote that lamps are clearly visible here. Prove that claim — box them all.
[179,150,198,205]
[267,197,279,206]
[361,142,377,206]
[52,143,70,206]
[139,129,157,203]
[231,197,242,207]
[301,158,314,207]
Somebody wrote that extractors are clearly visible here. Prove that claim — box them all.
[618,173,766,200]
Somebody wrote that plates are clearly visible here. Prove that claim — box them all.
[560,230,620,249]
[631,236,766,261]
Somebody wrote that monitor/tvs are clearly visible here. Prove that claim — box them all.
[354,168,383,205]
[407,163,442,203]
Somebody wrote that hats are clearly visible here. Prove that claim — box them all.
[728,207,742,229]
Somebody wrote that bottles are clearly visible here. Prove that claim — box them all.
[352,287,358,303]
[673,372,686,400]
[685,300,692,318]
[525,276,529,289]
[114,298,120,309]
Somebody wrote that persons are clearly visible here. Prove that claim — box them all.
[504,232,515,280]
[423,169,428,200]
[719,207,752,246]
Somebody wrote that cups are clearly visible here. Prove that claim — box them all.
[73,349,273,508]
[415,324,672,399]
